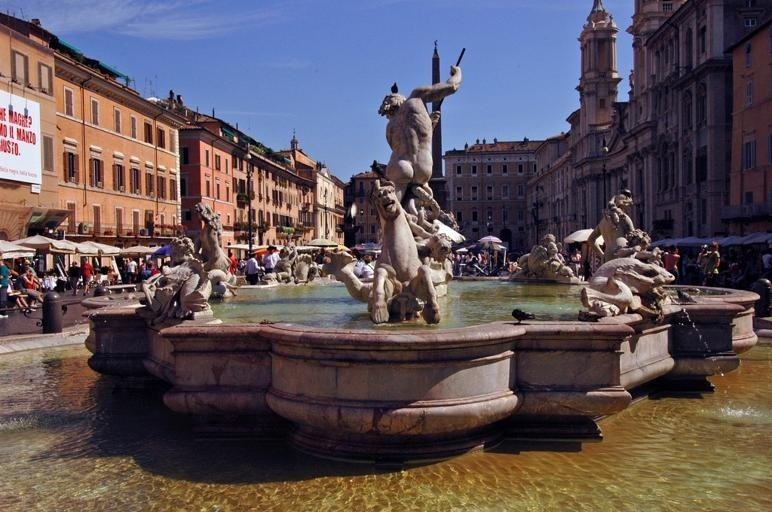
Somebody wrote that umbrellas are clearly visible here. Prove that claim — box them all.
[562,227,628,273]
[452,235,508,254]
[644,232,771,252]
[0,233,172,267]
[307,238,340,252]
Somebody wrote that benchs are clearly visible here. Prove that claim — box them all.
[106,283,136,294]
[11,278,42,306]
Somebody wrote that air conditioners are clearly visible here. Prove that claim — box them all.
[78,222,90,234]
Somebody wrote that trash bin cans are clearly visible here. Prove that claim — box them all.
[93,287,108,297]
[42,292,62,333]
[755,278,772,317]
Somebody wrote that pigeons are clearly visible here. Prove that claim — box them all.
[512,309,535,323]
[476,138,479,144]
[465,142,468,149]
[674,288,698,304]
[371,160,386,179]
[578,310,606,322]
[391,82,398,94]
[682,287,703,293]
[483,138,486,144]
[494,138,497,143]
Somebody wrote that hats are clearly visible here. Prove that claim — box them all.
[248,250,257,255]
[267,245,276,250]
[24,259,31,265]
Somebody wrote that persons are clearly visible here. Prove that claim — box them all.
[652,240,771,316]
[616,229,662,266]
[223,244,352,286]
[528,233,557,277]
[68,261,82,296]
[1,259,46,319]
[376,64,463,202]
[357,255,375,278]
[106,257,161,286]
[449,251,521,278]
[556,242,583,276]
[81,257,95,297]
[585,191,636,263]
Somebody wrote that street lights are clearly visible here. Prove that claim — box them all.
[242,151,255,254]
[601,136,610,213]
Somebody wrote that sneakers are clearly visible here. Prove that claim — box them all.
[83,291,90,296]
[0,314,9,318]
[22,307,36,313]
